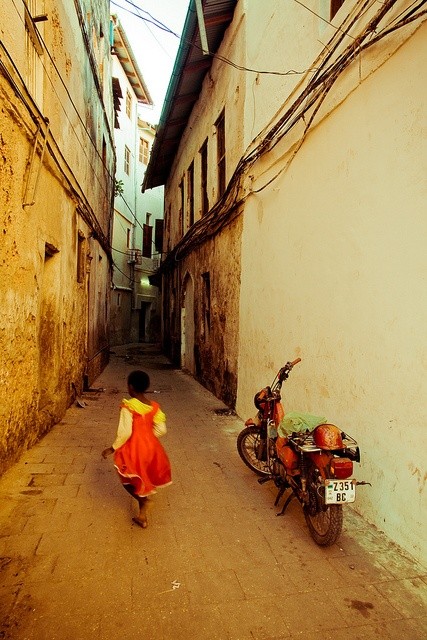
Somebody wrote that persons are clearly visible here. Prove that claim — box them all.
[101,369,174,529]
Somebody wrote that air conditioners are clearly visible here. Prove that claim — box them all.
[128,249,142,265]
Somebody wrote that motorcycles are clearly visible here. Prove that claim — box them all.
[236,358,372,548]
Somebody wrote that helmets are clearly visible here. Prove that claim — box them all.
[313,424,347,450]
